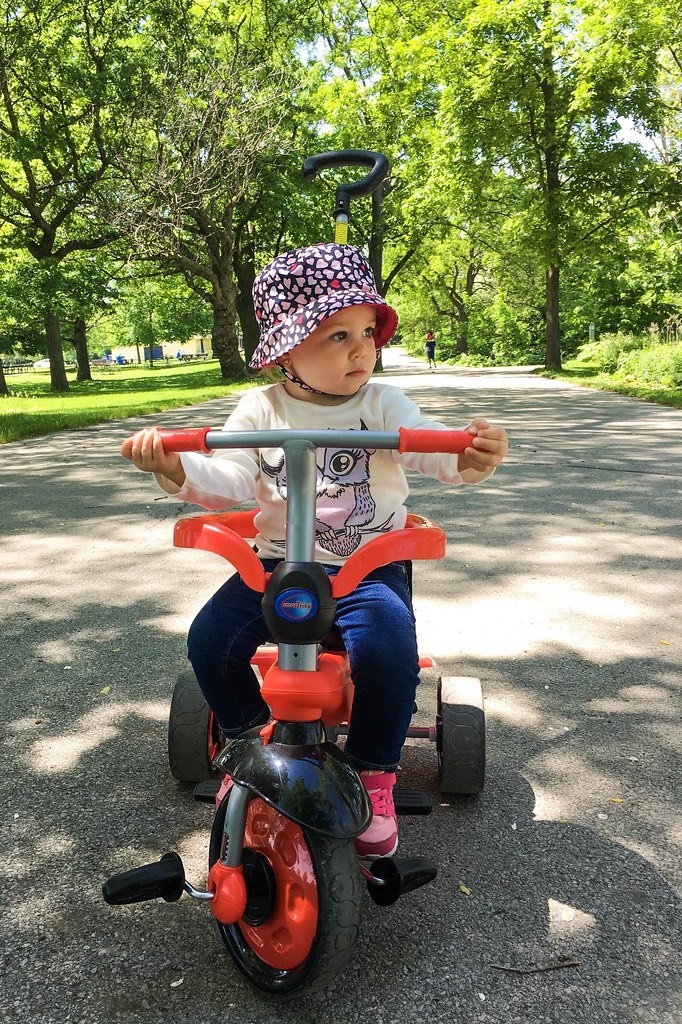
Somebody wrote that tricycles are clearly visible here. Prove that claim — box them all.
[100,149,490,1005]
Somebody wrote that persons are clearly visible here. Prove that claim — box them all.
[120,240,508,860]
[426,330,436,368]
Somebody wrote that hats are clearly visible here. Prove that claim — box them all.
[249,244,397,369]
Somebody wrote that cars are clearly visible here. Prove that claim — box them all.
[32,357,68,369]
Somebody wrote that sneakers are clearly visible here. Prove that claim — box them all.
[352,771,398,860]
[217,774,234,808]
